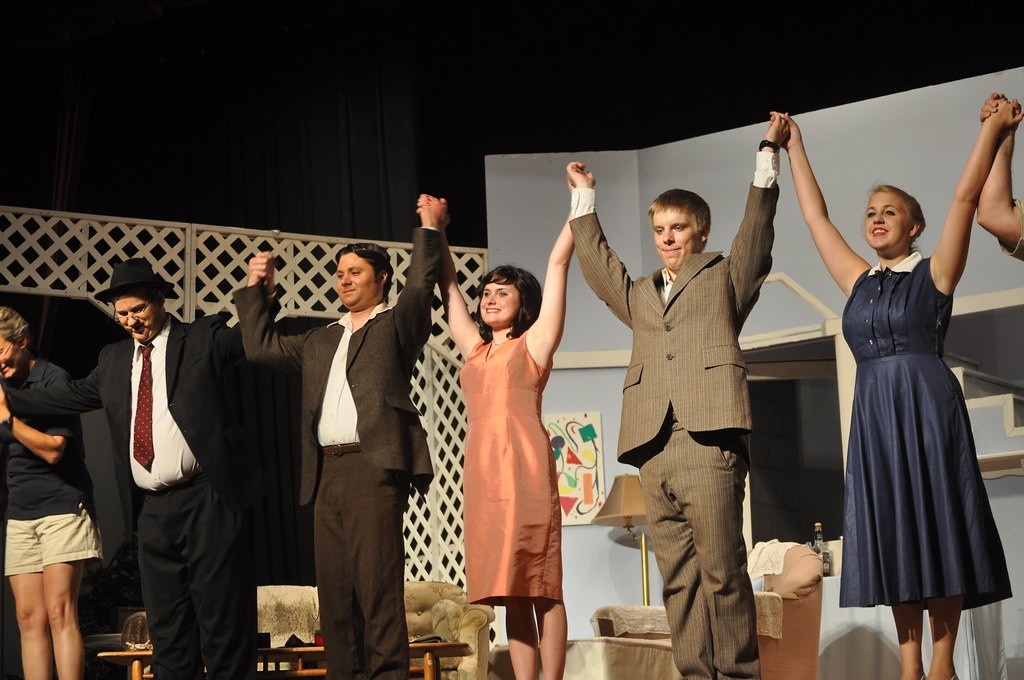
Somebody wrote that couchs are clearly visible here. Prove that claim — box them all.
[123,580,496,680]
[488,539,824,680]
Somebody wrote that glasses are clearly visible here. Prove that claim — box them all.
[113,297,153,325]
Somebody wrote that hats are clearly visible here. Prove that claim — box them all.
[94,258,175,302]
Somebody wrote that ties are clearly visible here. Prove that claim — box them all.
[134,343,154,467]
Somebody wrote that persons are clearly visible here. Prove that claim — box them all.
[977,91,1024,260]
[770,99,1024,680]
[0,251,287,680]
[415,170,576,680]
[0,302,102,680]
[232,192,447,680]
[564,111,789,680]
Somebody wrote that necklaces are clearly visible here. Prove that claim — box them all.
[492,343,500,346]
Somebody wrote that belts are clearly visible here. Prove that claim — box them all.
[323,443,361,456]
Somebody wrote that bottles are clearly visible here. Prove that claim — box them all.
[812,523,830,577]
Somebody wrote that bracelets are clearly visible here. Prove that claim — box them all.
[265,290,277,299]
[759,140,780,153]
[2,414,13,432]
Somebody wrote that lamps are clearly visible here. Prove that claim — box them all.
[590,474,653,606]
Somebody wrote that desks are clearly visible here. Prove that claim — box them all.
[98,641,474,680]
[751,576,1008,680]
